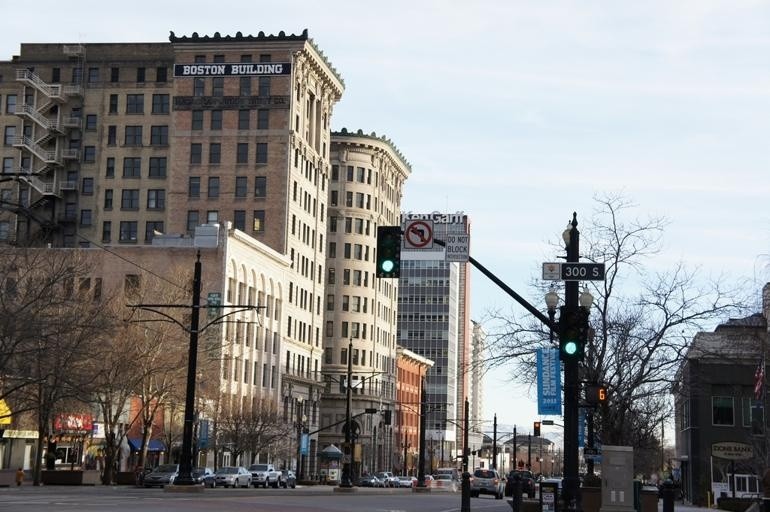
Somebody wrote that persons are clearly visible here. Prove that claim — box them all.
[16,467,25,487]
[318,471,327,485]
[43,447,57,470]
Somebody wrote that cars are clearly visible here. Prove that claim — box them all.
[425,468,457,492]
[143,464,179,488]
[358,472,399,487]
[173,467,216,488]
[399,477,418,488]
[272,470,296,488]
[214,467,252,488]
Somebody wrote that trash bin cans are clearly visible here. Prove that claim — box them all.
[539,481,557,512]
[319,474,326,484]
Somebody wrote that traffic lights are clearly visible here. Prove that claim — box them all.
[559,305,585,361]
[376,225,401,279]
[534,422,540,437]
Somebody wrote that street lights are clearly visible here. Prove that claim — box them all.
[293,395,307,480]
[543,219,593,511]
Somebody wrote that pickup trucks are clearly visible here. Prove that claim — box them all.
[248,464,282,488]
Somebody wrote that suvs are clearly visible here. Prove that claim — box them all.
[470,469,505,499]
[506,470,535,498]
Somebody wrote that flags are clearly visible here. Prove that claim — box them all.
[753,353,765,402]
